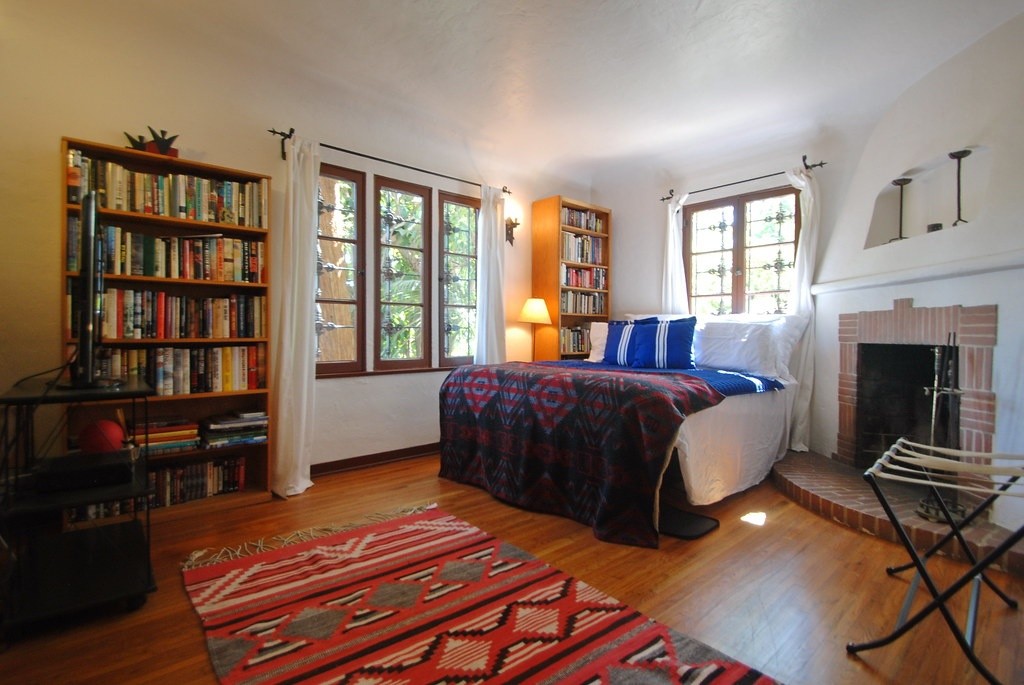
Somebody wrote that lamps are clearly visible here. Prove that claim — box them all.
[517,296,553,363]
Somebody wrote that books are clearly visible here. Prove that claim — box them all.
[561,206,605,353]
[67,148,268,524]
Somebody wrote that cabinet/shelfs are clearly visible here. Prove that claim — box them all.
[530,193,612,362]
[60,135,272,529]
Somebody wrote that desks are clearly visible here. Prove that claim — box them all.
[0,374,161,627]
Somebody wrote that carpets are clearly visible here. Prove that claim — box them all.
[179,503,783,685]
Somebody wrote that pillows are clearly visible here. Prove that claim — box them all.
[726,311,812,380]
[601,322,641,366]
[631,315,697,369]
[694,316,782,378]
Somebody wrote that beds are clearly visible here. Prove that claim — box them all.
[441,362,799,535]
